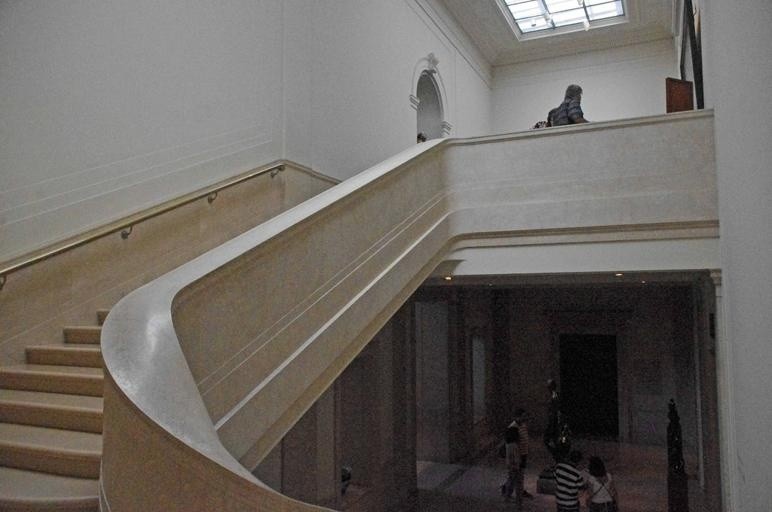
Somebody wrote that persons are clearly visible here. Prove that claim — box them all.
[495,378,620,512]
[548,84,590,127]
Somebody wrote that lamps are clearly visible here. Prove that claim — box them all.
[543,11,552,24]
[530,18,536,27]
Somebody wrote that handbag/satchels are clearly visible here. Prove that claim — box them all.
[498,445,506,458]
[585,498,593,507]
